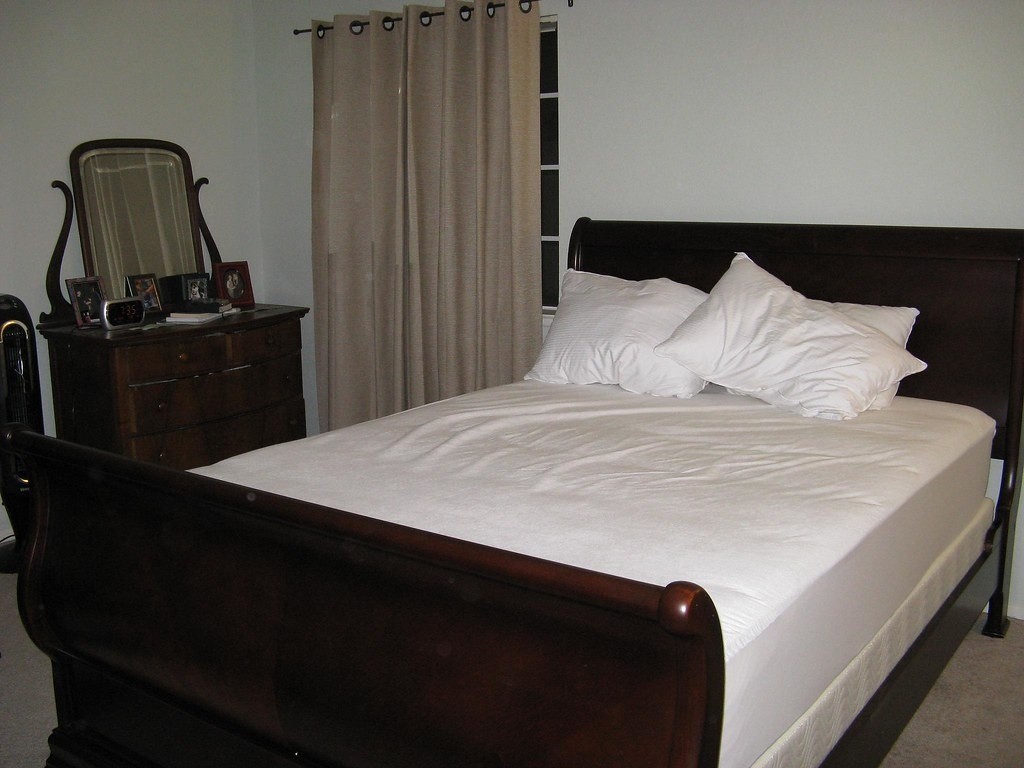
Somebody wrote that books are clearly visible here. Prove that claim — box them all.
[166,302,240,325]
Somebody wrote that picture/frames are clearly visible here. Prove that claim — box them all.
[64,275,109,330]
[124,273,167,318]
[181,272,211,301]
[213,260,256,307]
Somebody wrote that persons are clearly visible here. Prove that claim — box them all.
[76,286,102,322]
[227,272,243,298]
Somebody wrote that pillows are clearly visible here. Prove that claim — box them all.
[652,251,928,422]
[523,267,713,398]
[807,299,920,411]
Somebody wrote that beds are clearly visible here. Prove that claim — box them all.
[0,215,1024,768]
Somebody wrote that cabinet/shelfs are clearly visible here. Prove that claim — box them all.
[36,305,312,472]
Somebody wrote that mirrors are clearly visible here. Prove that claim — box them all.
[68,137,205,301]
